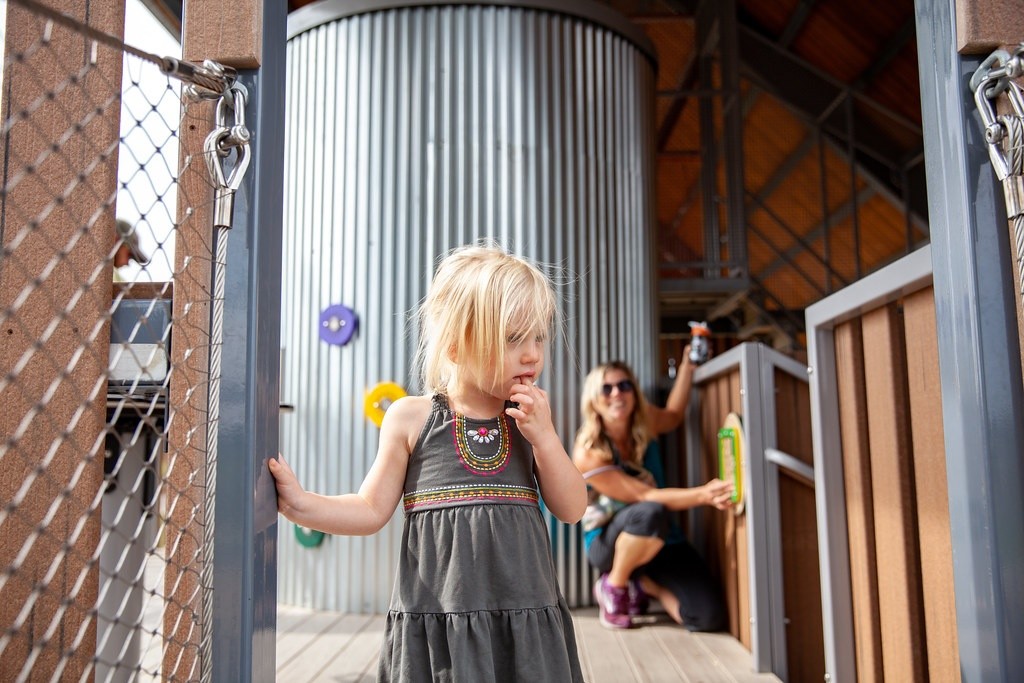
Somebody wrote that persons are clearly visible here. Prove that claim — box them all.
[571,344,732,633]
[267,242,587,683]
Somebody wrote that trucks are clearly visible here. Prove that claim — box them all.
[107,299,171,410]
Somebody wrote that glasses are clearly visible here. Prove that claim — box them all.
[603,380,633,394]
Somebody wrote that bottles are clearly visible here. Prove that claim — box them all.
[688,321,710,364]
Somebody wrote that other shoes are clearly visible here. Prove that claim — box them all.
[629,576,650,615]
[595,574,631,628]
